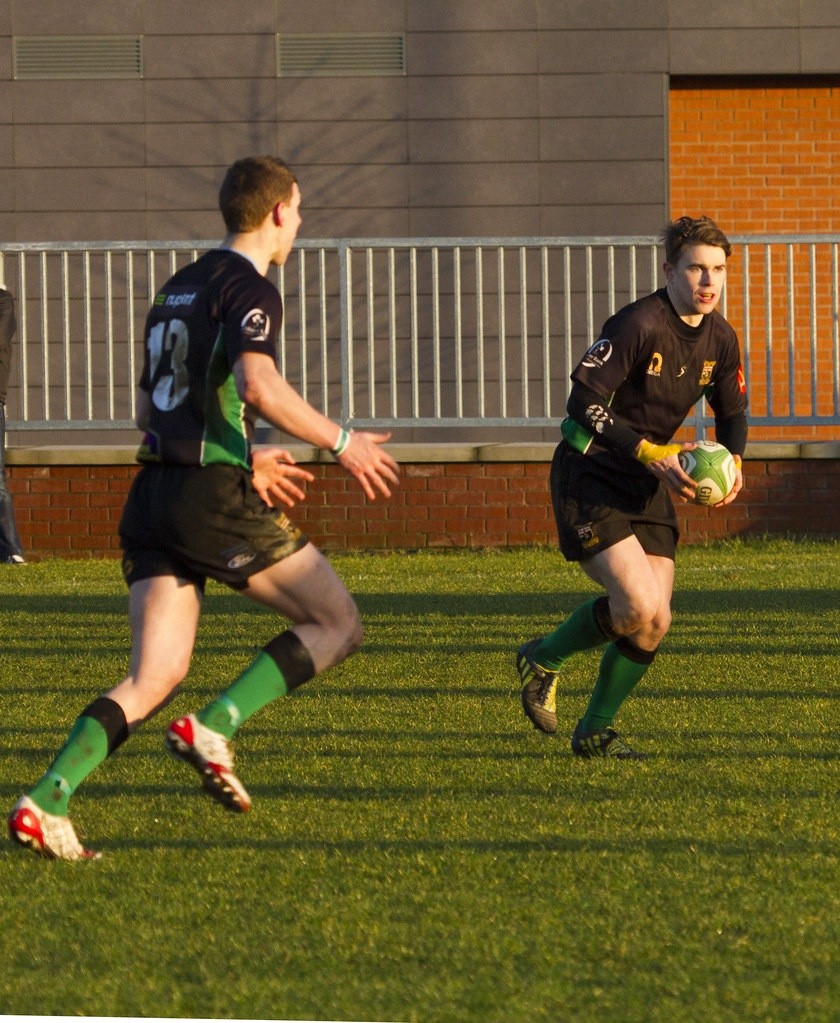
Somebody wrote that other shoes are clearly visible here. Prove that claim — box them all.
[3,554,28,566]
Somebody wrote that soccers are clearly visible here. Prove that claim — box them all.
[676,442,738,506]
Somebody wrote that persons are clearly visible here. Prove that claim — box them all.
[512,214,751,757]
[0,287,27,568]
[10,154,402,863]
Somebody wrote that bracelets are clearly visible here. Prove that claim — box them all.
[330,428,351,456]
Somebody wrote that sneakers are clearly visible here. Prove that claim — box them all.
[515,639,561,733]
[164,713,252,812]
[570,719,645,760]
[9,797,102,863]
